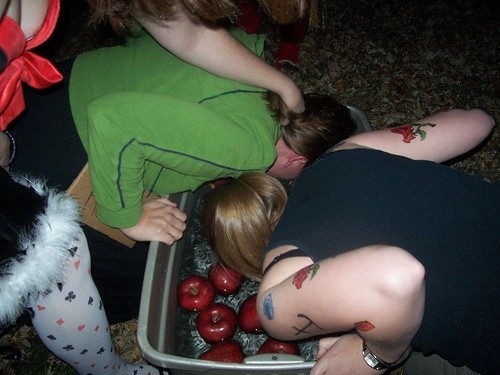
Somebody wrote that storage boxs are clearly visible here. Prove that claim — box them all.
[136,105,372,375]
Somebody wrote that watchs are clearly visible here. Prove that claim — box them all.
[362,339,412,371]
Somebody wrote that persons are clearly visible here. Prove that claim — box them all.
[199,108,500,375]
[0,0,308,120]
[0,167,175,375]
[0,16,360,249]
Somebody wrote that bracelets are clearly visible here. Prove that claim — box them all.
[3,131,15,167]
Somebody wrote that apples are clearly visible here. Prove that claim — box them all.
[198,339,245,363]
[237,292,264,334]
[208,261,245,295]
[257,337,300,355]
[195,303,237,343]
[178,275,215,312]
